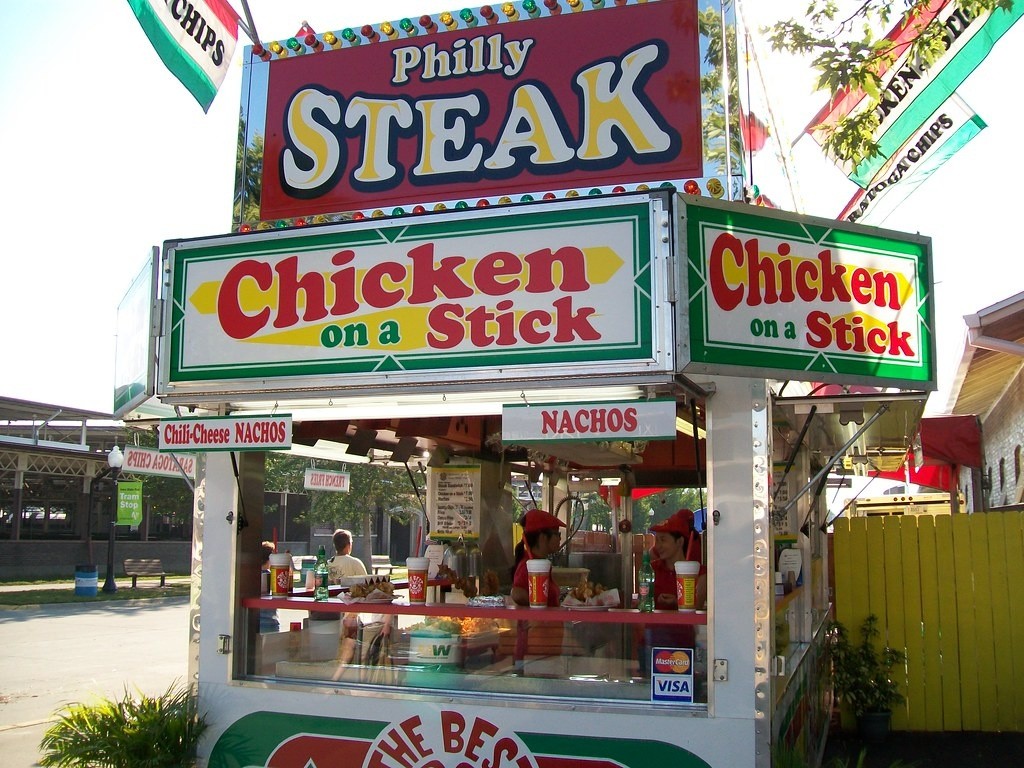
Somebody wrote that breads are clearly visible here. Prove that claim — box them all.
[424,616,497,634]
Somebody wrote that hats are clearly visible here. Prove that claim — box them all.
[676,509,694,521]
[523,509,566,532]
[649,517,689,538]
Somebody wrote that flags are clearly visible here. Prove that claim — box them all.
[807,0,1024,188]
[127,0,240,115]
[839,91,986,226]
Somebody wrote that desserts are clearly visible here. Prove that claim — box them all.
[348,577,396,598]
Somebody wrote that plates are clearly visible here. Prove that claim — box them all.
[340,596,394,603]
[561,603,615,611]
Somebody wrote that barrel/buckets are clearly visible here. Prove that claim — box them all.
[300,558,316,582]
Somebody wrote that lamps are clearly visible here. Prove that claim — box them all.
[981,474,991,492]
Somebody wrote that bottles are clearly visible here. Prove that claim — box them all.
[314,545,330,602]
[637,549,655,613]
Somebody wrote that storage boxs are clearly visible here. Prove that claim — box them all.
[569,530,612,551]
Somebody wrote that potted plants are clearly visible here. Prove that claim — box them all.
[824,613,908,747]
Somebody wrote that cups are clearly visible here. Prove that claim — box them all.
[406,556,431,605]
[674,560,700,613]
[526,559,551,609]
[268,553,290,598]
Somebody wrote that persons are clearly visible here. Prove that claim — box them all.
[258,541,279,632]
[638,509,707,672]
[509,509,567,654]
[325,528,367,584]
[332,612,395,685]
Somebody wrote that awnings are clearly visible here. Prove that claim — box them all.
[868,415,982,493]
[773,393,925,472]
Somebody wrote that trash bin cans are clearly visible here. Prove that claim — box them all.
[74,565,99,597]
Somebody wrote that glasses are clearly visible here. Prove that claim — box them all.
[552,532,561,537]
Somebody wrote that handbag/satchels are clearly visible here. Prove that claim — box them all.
[363,632,398,685]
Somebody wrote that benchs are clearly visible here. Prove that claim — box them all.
[122,558,175,590]
[293,555,318,571]
[371,554,399,575]
